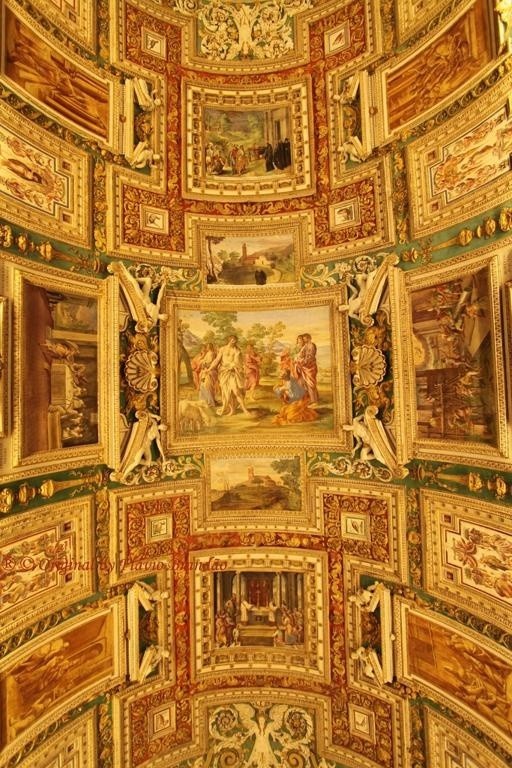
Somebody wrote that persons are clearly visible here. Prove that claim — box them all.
[122,413,169,483]
[437,627,512,741]
[347,581,379,608]
[137,580,172,607]
[336,269,379,323]
[351,648,373,679]
[139,80,162,112]
[190,334,319,425]
[411,280,499,442]
[335,141,364,166]
[3,636,79,726]
[207,138,290,175]
[330,78,352,103]
[209,592,304,647]
[118,259,168,327]
[35,332,96,451]
[132,147,162,170]
[340,414,384,466]
[146,645,170,678]
[238,712,286,768]
